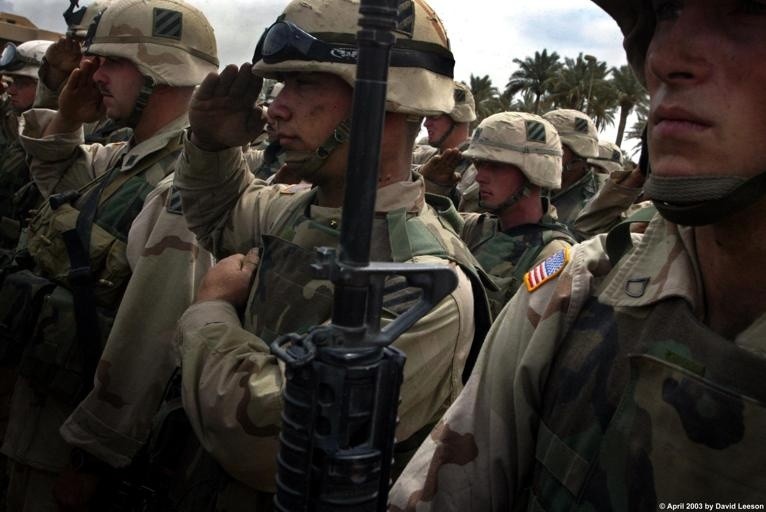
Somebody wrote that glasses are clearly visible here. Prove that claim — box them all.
[0,42,42,70]
[251,19,455,78]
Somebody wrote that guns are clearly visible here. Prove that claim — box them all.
[269,0,458,512]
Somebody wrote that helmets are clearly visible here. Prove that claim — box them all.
[81,0,220,89]
[461,111,563,191]
[248,0,456,118]
[543,108,601,159]
[453,81,477,123]
[0,39,58,80]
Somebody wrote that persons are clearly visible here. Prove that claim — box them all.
[0,1,763,511]
